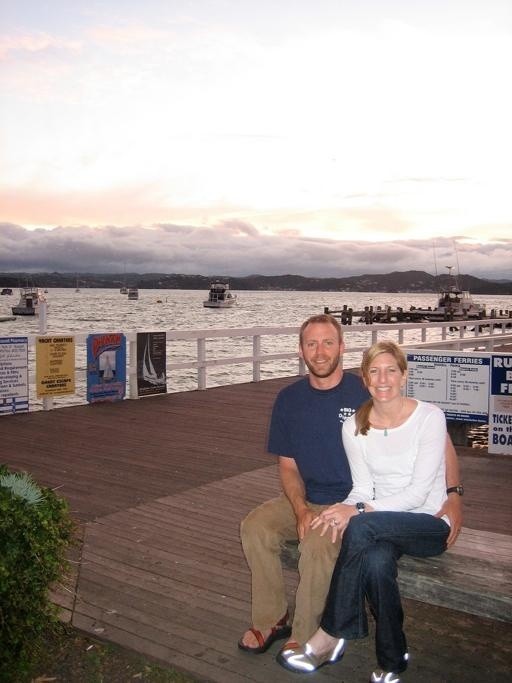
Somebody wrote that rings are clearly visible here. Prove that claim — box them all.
[332,518,337,525]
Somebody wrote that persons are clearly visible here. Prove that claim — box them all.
[238,313,466,654]
[279,340,452,681]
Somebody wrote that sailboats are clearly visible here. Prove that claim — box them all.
[101,353,115,382]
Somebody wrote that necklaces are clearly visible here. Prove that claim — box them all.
[384,427,389,437]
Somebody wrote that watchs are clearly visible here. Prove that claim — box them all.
[356,501,365,514]
[447,485,464,495]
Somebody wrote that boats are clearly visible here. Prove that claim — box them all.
[11,288,50,316]
[203,278,237,308]
[1,288,13,295]
[410,265,486,317]
[120,286,138,300]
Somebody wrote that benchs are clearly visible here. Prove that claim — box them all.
[280,526,512,620]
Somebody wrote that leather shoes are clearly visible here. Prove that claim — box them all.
[371,650,408,683]
[276,637,345,673]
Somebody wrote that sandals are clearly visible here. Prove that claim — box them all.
[237,608,293,654]
[281,633,301,649]
